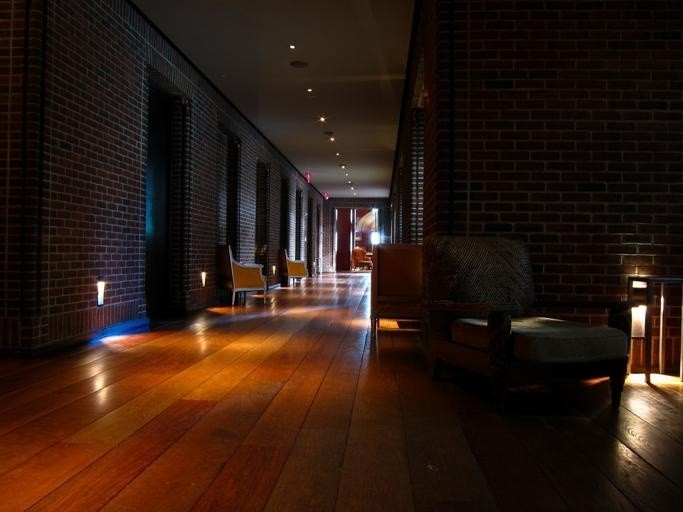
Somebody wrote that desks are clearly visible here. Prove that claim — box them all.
[626,272,681,384]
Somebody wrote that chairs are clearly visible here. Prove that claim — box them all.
[433,237,629,430]
[369,241,421,356]
[279,248,308,286]
[216,243,269,305]
[351,246,372,270]
